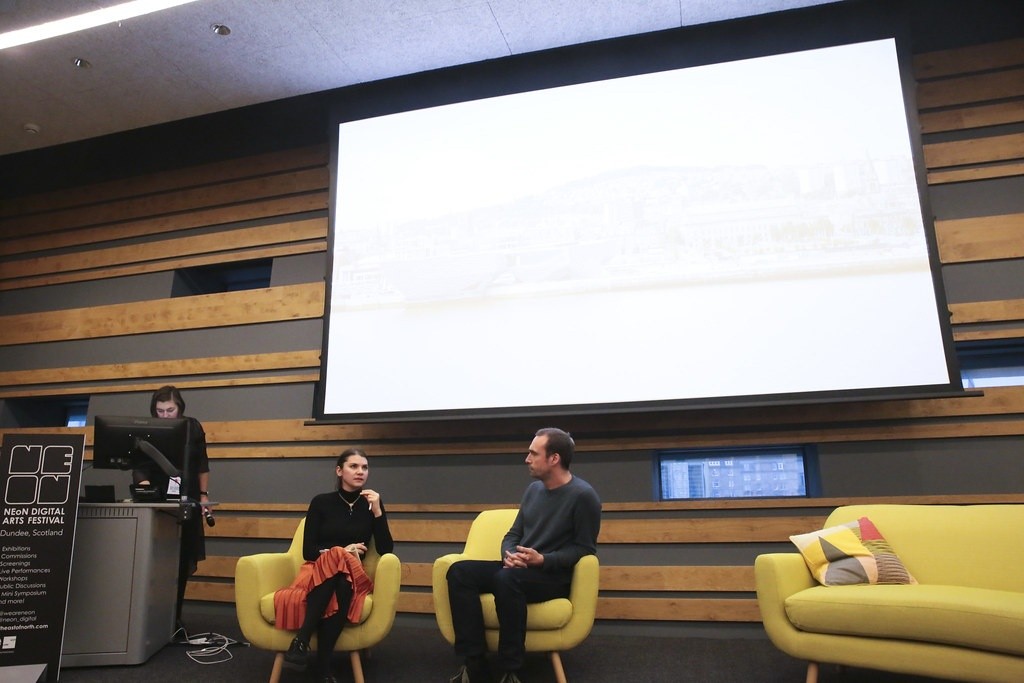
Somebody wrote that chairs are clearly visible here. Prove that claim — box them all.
[434,510,603,683]
[231,515,403,682]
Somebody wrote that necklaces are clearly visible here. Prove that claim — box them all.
[339,490,361,515]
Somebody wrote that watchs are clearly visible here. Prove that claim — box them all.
[200,491,209,496]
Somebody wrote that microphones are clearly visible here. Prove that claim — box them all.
[203,507,216,527]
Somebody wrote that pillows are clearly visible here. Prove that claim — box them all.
[787,515,915,585]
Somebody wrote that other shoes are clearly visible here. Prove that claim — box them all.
[283,635,310,671]
[450,669,523,683]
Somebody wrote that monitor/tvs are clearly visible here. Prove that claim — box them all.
[93,416,192,499]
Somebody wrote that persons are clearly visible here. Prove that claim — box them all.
[130,385,210,637]
[283,448,394,683]
[446,428,602,683]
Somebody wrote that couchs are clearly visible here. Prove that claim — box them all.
[755,502,1024,682]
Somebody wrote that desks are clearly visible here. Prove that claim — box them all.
[60,499,221,668]
